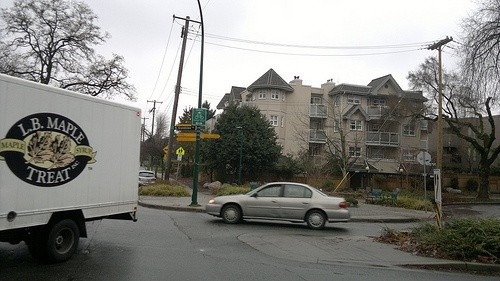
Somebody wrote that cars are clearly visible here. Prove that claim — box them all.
[205,182,351,229]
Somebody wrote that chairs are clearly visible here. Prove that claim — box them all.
[366,189,382,203]
[382,193,398,207]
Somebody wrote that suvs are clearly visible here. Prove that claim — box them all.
[139,170,156,187]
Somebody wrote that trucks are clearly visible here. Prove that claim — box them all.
[0,74,141,262]
